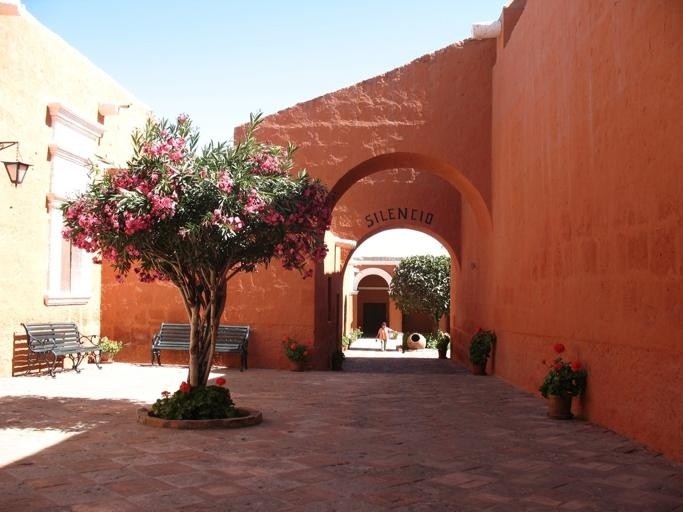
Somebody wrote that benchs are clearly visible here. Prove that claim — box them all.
[148,322,253,371]
[20,321,102,378]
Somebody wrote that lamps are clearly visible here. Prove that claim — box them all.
[0,139,33,188]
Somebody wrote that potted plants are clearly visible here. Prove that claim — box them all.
[342,336,352,351]
[330,360,343,372]
[98,336,122,362]
[434,332,450,359]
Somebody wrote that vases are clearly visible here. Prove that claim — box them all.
[545,392,575,421]
[469,360,485,375]
[288,359,303,371]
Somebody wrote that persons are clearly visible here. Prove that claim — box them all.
[374,321,397,352]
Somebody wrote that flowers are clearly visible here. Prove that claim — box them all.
[332,342,345,361]
[282,335,312,361]
[538,343,586,398]
[469,327,494,364]
